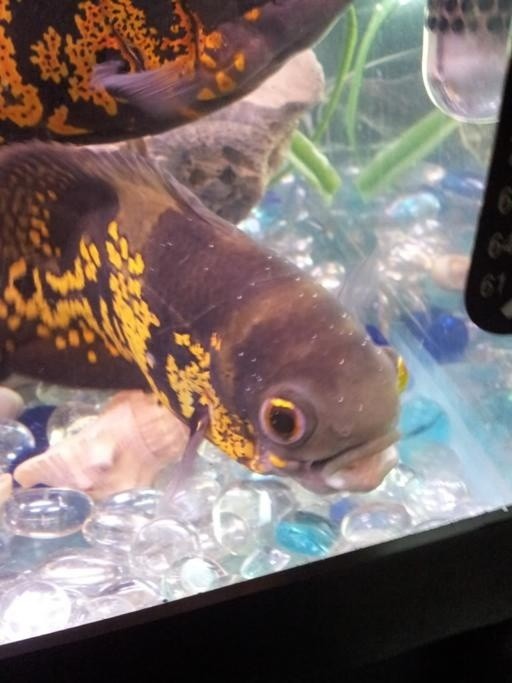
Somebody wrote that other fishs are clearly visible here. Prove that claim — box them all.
[0,137,409,494]
[0,0,352,144]
[145,99,308,225]
